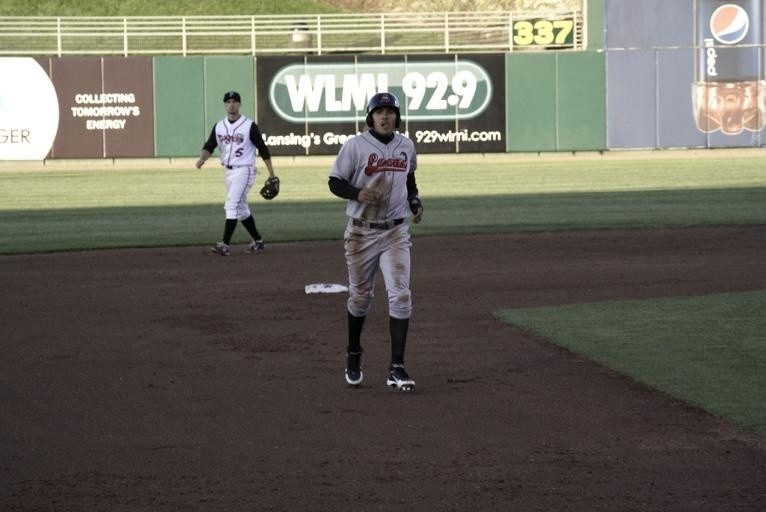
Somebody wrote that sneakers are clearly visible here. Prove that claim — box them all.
[344,345,364,385]
[210,240,231,256]
[244,239,266,254]
[386,363,415,392]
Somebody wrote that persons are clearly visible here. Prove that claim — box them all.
[327,91,426,392]
[195,92,279,257]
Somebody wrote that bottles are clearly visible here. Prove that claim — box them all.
[693,1,765,135]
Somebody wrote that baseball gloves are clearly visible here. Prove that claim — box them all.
[259,177,279,199]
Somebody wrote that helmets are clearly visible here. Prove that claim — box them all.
[366,93,400,130]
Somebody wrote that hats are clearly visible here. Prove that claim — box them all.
[223,91,241,103]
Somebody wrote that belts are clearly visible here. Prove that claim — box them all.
[221,163,239,169]
[353,218,404,231]
